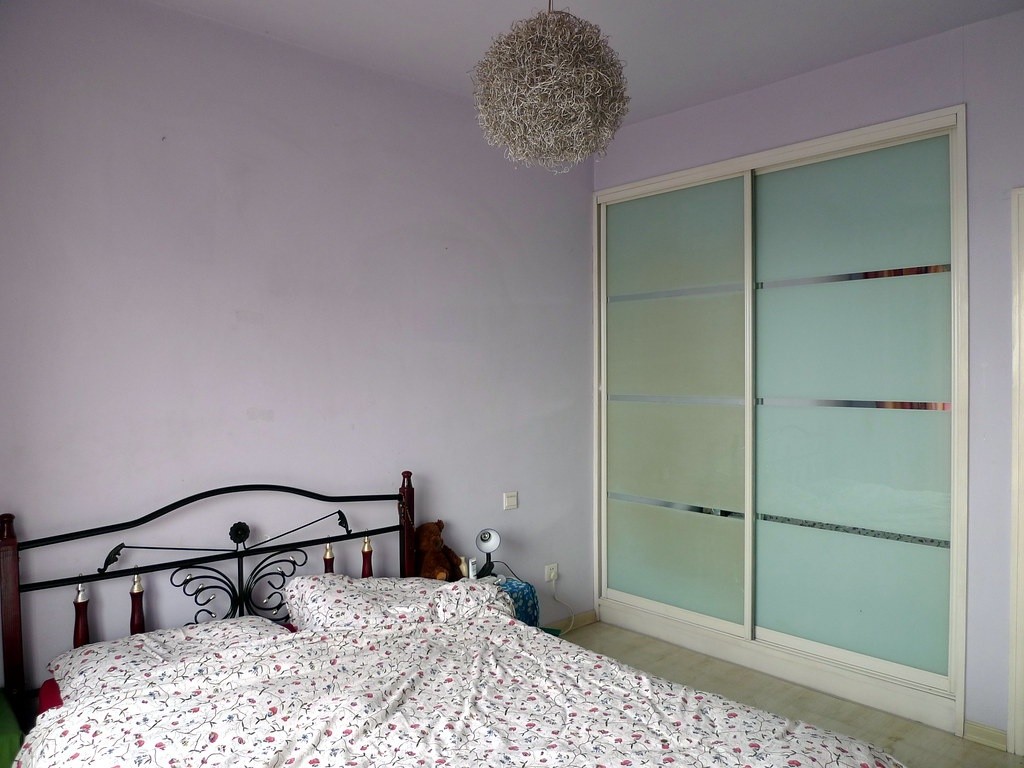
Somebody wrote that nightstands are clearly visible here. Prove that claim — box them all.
[479,573,541,629]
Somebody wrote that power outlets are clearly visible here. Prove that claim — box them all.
[545,562,558,581]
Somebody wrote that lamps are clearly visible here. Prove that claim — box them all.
[474,528,501,579]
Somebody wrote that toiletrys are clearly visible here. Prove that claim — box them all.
[468,558,477,580]
[459,556,467,577]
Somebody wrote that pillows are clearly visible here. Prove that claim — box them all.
[45,615,291,701]
[282,573,449,632]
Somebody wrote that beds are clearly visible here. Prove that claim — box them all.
[0,471,906,768]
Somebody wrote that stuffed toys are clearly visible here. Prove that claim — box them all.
[414,518,464,583]
